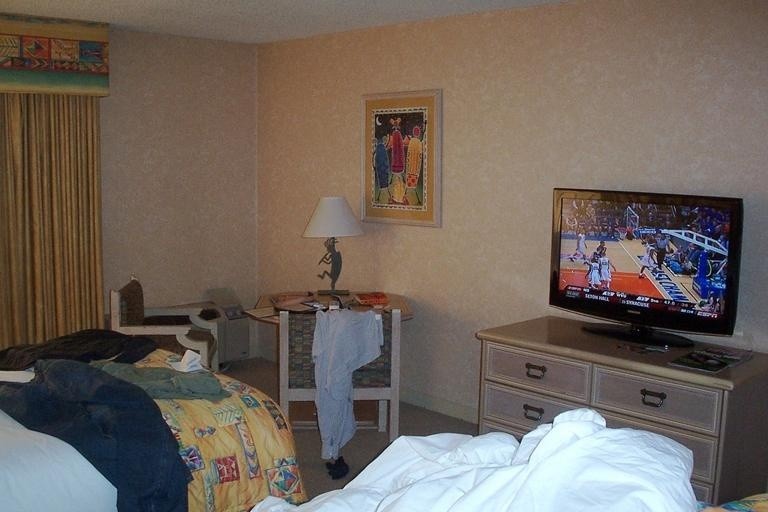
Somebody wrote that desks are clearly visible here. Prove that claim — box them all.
[256,293,414,326]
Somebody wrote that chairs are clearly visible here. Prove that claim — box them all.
[278,308,402,442]
[110,279,226,372]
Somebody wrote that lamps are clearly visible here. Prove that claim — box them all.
[302,197,362,295]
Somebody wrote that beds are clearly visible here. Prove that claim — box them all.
[254,408,768,512]
[0,329,308,512]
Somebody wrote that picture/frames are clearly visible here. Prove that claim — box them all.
[359,88,441,229]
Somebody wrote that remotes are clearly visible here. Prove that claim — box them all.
[329,300,339,310]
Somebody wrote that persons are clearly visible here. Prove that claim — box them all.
[561,198,730,318]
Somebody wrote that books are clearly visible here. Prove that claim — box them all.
[351,292,391,306]
[666,346,754,375]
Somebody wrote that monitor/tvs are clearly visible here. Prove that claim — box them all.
[547,187,744,346]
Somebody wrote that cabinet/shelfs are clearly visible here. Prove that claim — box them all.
[475,316,768,505]
